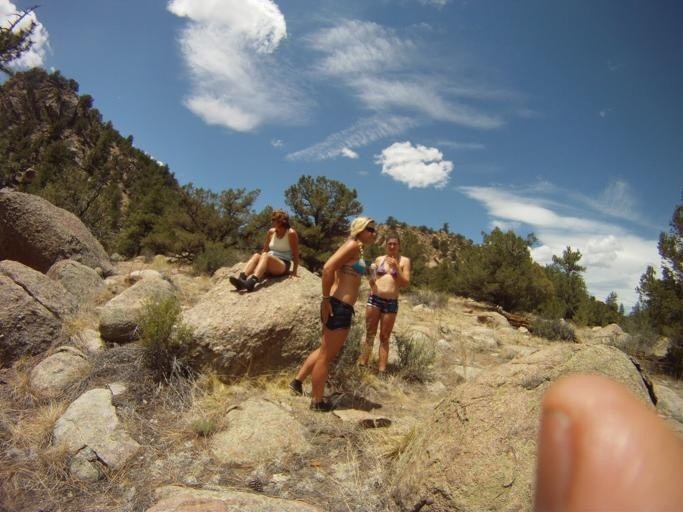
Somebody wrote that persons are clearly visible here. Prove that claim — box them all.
[535,374,683,512]
[229,210,300,292]
[358,234,411,372]
[288,216,376,411]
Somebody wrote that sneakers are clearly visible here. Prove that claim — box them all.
[378,370,386,382]
[309,399,330,411]
[289,378,302,396]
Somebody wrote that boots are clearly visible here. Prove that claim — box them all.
[239,275,258,291]
[230,273,247,290]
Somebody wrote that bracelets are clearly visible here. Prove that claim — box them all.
[320,296,330,301]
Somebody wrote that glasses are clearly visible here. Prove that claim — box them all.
[272,218,277,221]
[365,227,373,233]
